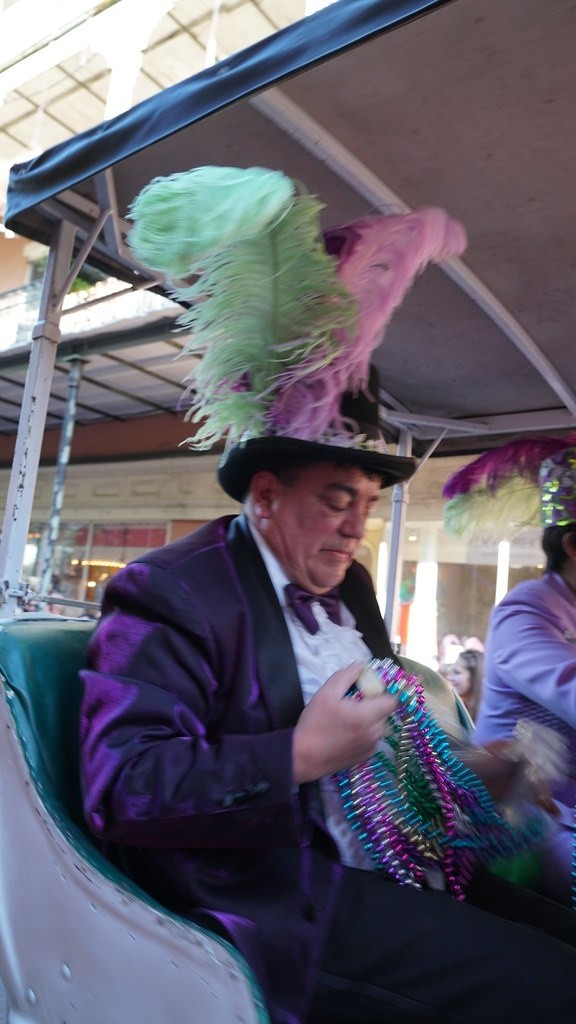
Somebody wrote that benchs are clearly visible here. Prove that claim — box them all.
[0,612,475,1024]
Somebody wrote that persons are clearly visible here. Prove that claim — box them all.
[471,520,576,884]
[440,649,485,725]
[79,435,576,1024]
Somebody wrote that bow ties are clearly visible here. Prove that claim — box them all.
[283,581,344,634]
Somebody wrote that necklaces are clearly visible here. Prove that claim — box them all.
[332,658,541,904]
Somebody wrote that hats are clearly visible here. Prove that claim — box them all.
[538,448,576,526]
[218,359,416,502]
